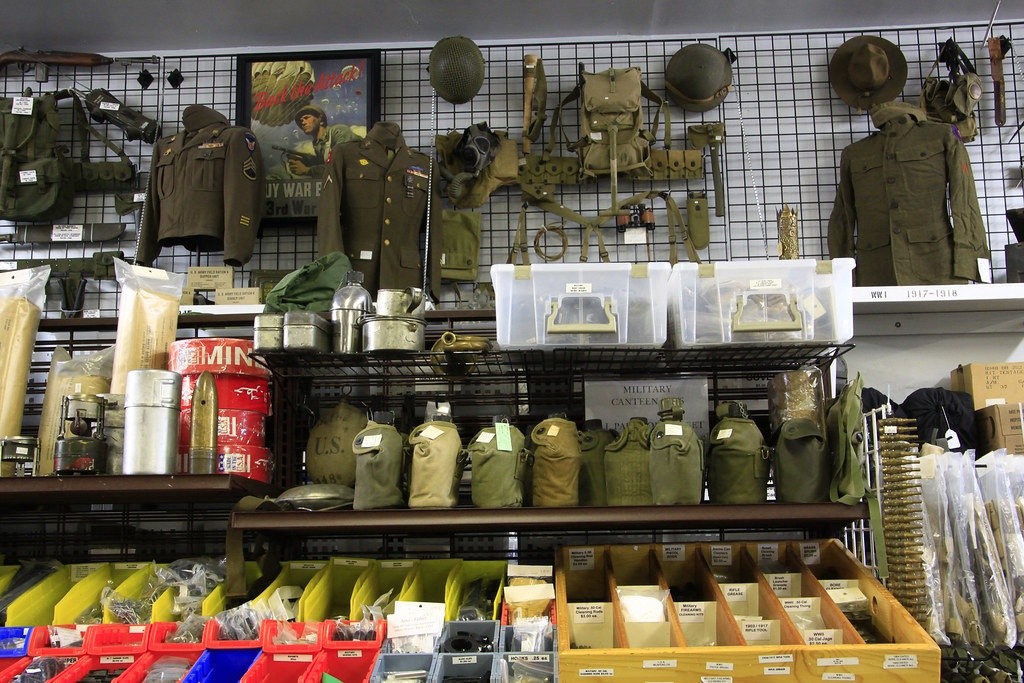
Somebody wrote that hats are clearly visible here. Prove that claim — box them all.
[828,35,908,110]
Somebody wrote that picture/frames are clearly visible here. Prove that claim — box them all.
[235,48,381,228]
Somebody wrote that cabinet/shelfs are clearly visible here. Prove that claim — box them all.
[226,348,870,528]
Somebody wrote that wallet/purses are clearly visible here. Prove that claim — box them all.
[80,118,136,192]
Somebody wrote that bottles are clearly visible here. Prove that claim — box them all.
[330,271,373,353]
[547,412,566,421]
[432,414,450,422]
[629,417,647,424]
[728,402,748,417]
[585,419,602,429]
[492,415,510,426]
[657,398,685,421]
[373,410,394,425]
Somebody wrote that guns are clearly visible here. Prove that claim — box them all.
[271,144,323,167]
[0,47,161,83]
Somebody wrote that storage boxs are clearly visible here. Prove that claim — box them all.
[432,653,498,683]
[496,651,559,683]
[0,557,508,627]
[381,634,441,653]
[667,258,855,364]
[490,262,672,369]
[501,598,557,625]
[441,620,500,652]
[950,362,1024,411]
[499,626,558,652]
[370,652,439,683]
[555,538,941,683]
[0,647,380,683]
[976,403,1024,456]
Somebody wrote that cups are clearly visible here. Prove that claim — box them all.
[376,287,426,321]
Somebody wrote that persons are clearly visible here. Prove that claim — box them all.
[312,123,440,303]
[824,105,987,289]
[141,104,263,266]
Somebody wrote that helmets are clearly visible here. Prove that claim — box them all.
[293,104,327,131]
[666,44,733,113]
[430,36,485,104]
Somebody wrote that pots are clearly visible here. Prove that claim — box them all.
[352,317,430,352]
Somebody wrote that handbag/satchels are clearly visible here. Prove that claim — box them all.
[831,375,872,498]
[558,67,663,173]
[352,418,830,510]
[1,94,78,224]
[430,208,480,282]
